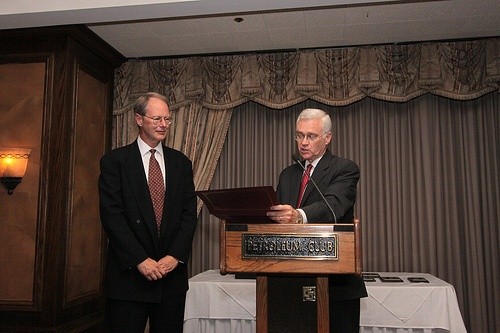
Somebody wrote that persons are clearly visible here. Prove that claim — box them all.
[263,109,368,333]
[97,92,197,333]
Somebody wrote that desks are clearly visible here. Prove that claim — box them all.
[182,269,467,333]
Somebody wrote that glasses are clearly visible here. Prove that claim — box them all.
[294,132,326,142]
[142,114,173,125]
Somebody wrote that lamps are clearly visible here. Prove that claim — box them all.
[0,148,32,195]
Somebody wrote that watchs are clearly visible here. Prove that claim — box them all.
[295,210,302,224]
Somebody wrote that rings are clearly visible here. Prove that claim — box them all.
[279,217,283,221]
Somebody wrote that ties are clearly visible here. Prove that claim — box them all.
[296,164,313,209]
[148,149,165,240]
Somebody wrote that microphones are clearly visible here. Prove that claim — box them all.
[292,154,337,224]
